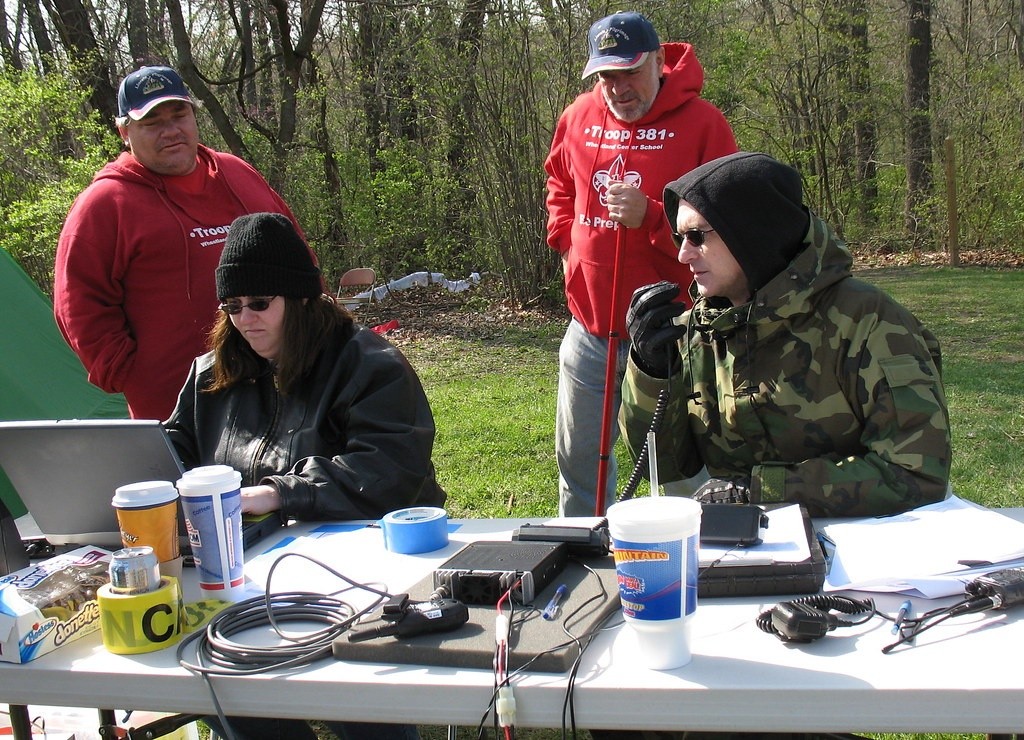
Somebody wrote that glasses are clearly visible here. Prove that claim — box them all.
[217,294,276,315]
[670,229,715,250]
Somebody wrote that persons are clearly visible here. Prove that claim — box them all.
[166,213,448,740]
[590,151,952,740]
[52,67,339,421]
[545,10,737,518]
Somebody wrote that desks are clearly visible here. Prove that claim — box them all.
[0,494,1023,735]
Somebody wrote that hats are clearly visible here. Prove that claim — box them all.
[580,10,660,81]
[118,66,195,121]
[215,212,324,300]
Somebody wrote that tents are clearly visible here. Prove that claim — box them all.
[0,245,161,540]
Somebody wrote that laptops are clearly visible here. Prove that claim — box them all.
[0,419,289,568]
[697,506,827,598]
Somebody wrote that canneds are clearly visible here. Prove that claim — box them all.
[109,546,160,595]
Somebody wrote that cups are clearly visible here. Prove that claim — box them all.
[175,465,246,599]
[607,496,703,671]
[109,481,180,563]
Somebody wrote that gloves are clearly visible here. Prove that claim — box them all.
[625,280,688,375]
[690,476,750,506]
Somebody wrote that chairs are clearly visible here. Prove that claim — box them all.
[333,268,392,334]
